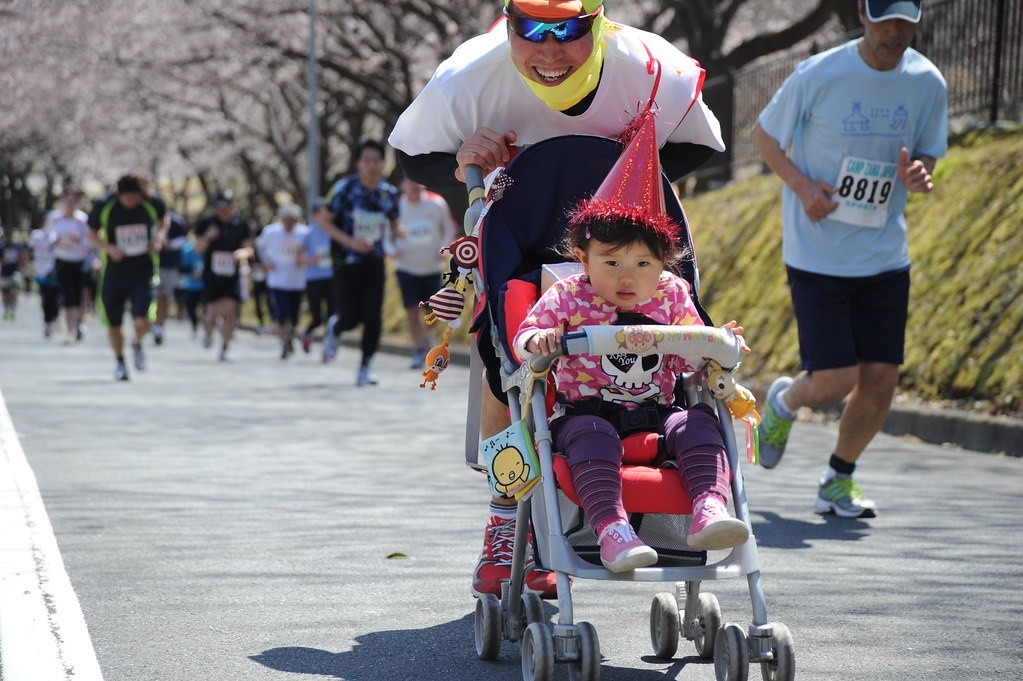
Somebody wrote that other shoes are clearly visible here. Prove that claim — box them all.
[322,315,340,363]
[280,339,293,359]
[358,368,377,385]
[220,346,227,360]
[203,329,212,347]
[131,340,145,369]
[115,363,128,380]
[412,333,437,368]
[299,331,312,352]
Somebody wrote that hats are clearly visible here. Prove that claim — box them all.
[865,0,923,23]
[569,96,680,244]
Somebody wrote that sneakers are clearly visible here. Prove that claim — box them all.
[815,478,877,519]
[756,377,797,469]
[525,520,574,599]
[599,524,658,573]
[471,515,525,599]
[687,498,749,551]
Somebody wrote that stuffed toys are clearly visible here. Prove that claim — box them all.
[705,360,761,424]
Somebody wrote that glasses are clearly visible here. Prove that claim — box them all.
[503,7,602,43]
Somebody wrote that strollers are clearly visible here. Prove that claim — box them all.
[462,135,800,681]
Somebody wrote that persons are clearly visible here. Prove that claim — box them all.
[386,1,726,601]
[512,215,751,572]
[749,0,949,518]
[0,137,454,386]
